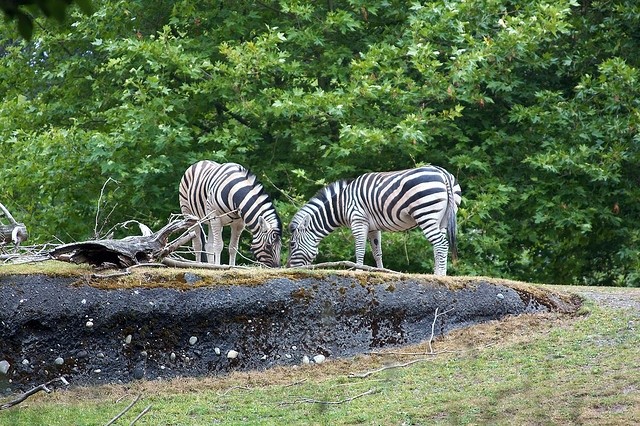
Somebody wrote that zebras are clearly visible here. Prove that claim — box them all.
[288,165,462,277]
[179,160,283,268]
